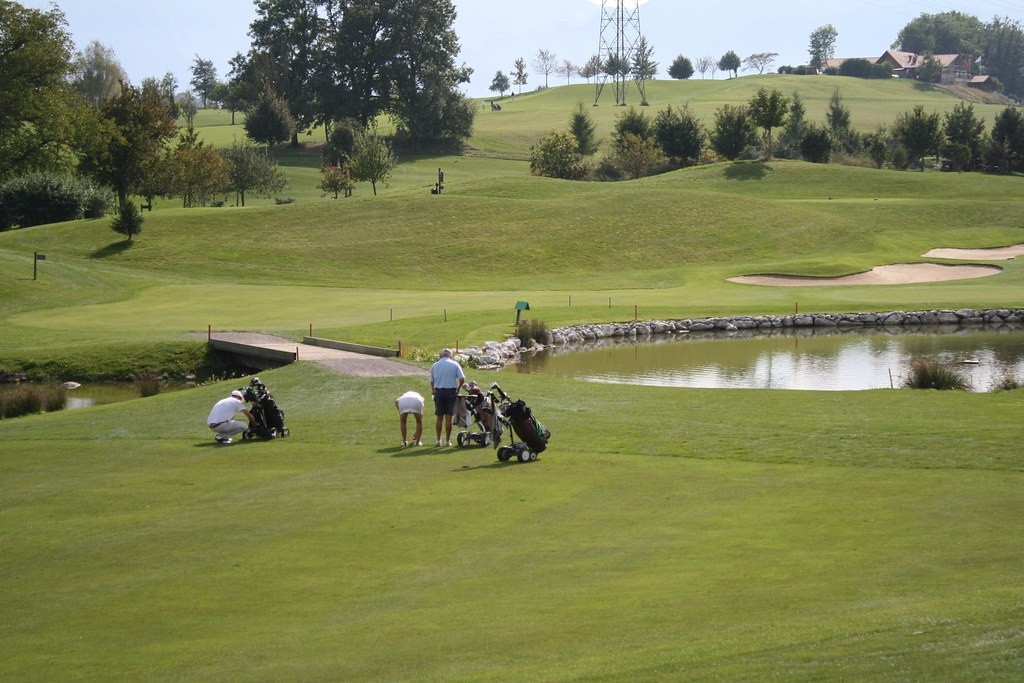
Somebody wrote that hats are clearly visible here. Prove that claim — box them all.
[231,390,243,398]
[441,348,453,356]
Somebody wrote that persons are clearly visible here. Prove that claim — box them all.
[430,348,465,446]
[207,390,257,444]
[396,391,425,446]
[492,104,501,111]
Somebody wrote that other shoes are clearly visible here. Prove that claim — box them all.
[446,441,452,446]
[403,441,408,447]
[415,442,423,446]
[214,434,233,443]
[435,441,442,446]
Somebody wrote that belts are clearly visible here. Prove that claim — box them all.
[210,420,228,428]
